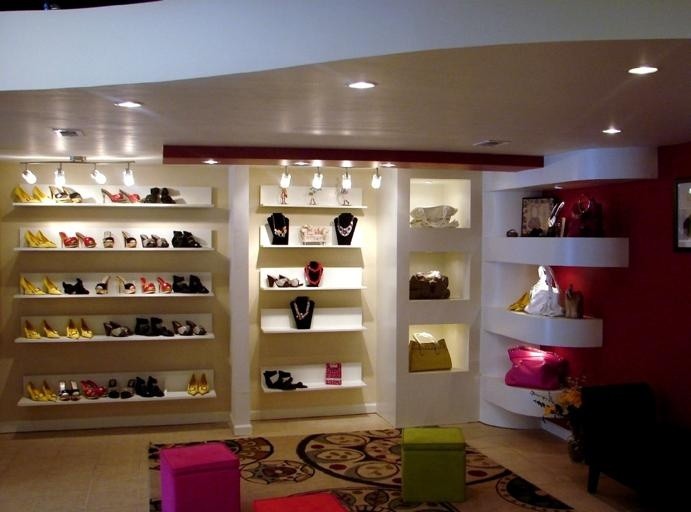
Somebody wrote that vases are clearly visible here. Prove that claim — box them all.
[567,441,584,463]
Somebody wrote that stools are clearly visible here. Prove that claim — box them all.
[401,429,468,503]
[159,442,239,511]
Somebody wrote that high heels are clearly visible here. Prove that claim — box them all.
[26,373,209,400]
[19,274,211,294]
[24,317,206,338]
[266,273,303,287]
[24,229,202,247]
[12,184,177,204]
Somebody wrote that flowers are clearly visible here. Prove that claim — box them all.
[529,375,585,441]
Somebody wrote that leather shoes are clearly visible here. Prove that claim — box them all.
[509,292,529,312]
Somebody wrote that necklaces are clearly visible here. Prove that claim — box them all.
[293,301,311,322]
[270,216,289,240]
[337,217,357,239]
[303,260,323,287]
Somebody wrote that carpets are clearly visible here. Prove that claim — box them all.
[149,426,579,512]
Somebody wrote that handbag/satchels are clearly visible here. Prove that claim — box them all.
[409,331,452,372]
[525,265,564,319]
[504,345,566,391]
[568,192,604,235]
[410,273,449,299]
[410,205,459,229]
[565,284,583,318]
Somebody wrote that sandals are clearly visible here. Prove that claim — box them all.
[264,368,308,392]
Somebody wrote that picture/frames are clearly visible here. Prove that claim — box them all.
[673,176,691,254]
